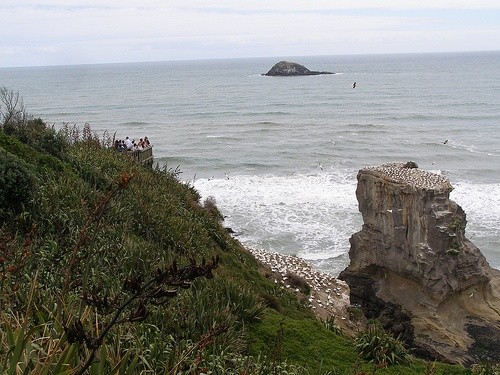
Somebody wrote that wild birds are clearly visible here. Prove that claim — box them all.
[442,140,448,145]
[319,163,323,170]
[331,139,336,146]
[352,82,356,88]
[208,176,214,181]
[224,172,229,179]
[432,161,436,165]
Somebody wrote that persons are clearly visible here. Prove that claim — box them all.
[111,136,153,153]
